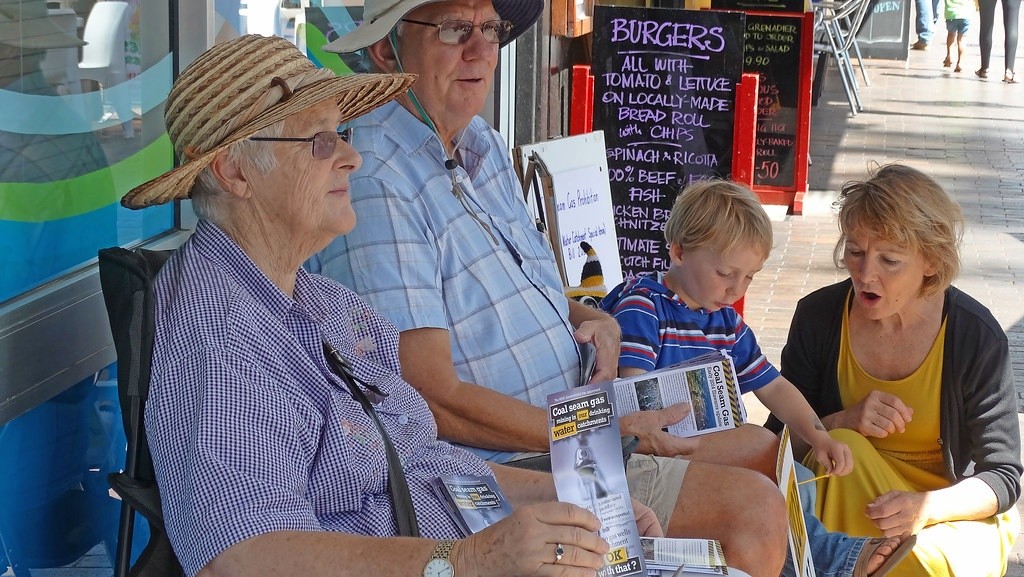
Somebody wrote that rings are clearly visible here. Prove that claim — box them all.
[552,542,565,565]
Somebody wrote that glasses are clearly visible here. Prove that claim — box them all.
[249,127,355,160]
[401,19,514,45]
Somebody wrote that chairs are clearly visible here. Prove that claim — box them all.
[98,244,186,577]
[814,0,872,116]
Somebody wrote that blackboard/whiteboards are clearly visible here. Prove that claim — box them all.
[699,6,817,195]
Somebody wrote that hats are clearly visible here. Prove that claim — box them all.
[119,33,419,211]
[321,0,546,53]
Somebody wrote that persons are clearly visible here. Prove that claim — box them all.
[598,169,919,576]
[912,0,1024,82]
[120,35,667,576]
[303,0,790,576]
[762,162,1024,577]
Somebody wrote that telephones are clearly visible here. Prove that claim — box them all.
[570,5,759,322]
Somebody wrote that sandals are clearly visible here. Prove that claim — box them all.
[851,530,917,577]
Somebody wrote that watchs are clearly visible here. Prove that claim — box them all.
[422,537,458,576]
